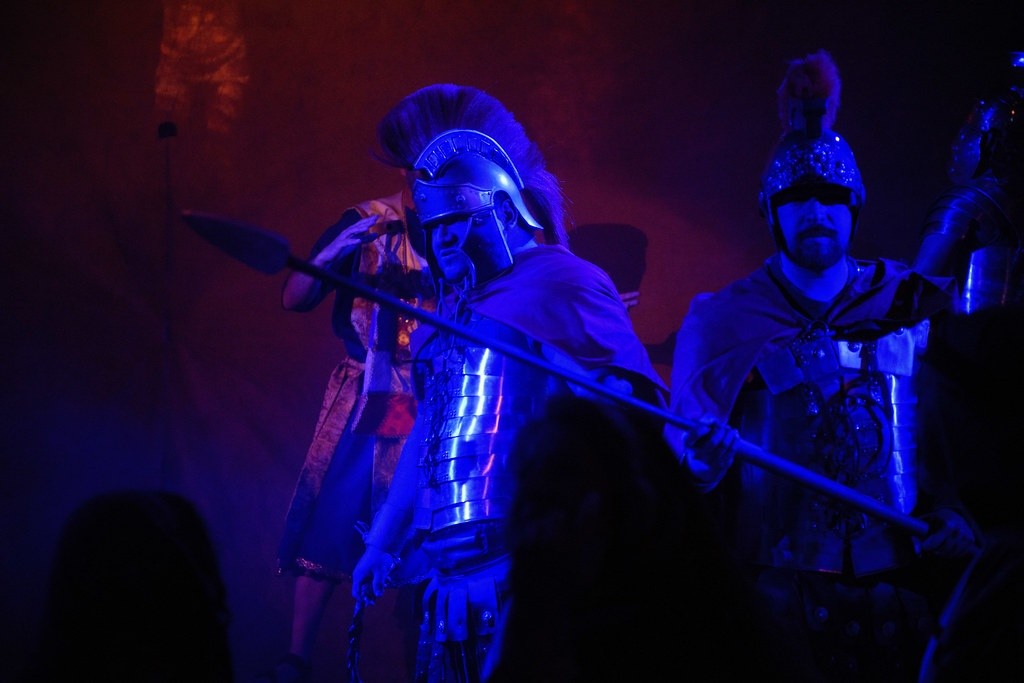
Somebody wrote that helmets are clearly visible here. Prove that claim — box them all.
[759,128,867,248]
[404,129,545,278]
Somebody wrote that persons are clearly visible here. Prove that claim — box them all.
[344,146,676,682]
[659,134,1005,683]
[278,164,446,682]
